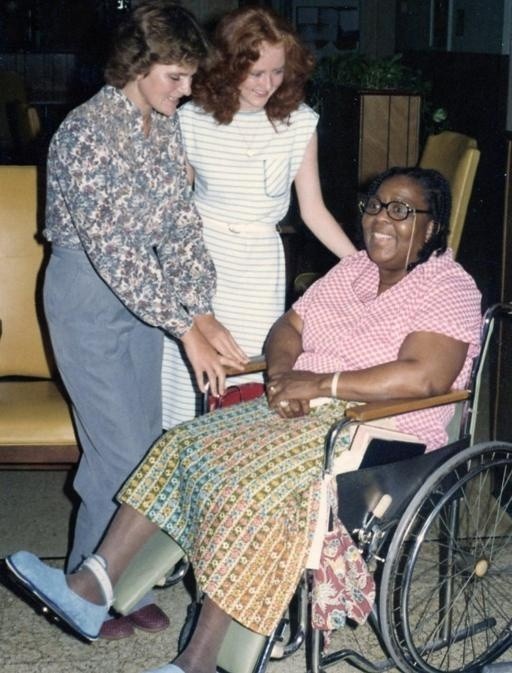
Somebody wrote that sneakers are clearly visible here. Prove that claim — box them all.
[127,605,170,632]
[150,663,182,673]
[6,549,107,643]
[98,618,135,640]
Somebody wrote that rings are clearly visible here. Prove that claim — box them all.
[269,385,276,394]
[279,399,288,408]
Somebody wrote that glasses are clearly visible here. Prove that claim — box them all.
[360,196,430,220]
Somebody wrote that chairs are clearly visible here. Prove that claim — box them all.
[293,130,482,293]
[0,163,82,474]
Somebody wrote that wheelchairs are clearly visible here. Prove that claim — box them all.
[149,301,512,673]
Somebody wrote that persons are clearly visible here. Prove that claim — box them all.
[6,162,484,672]
[37,0,251,640]
[153,4,359,429]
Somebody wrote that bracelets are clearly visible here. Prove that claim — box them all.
[331,370,342,398]
